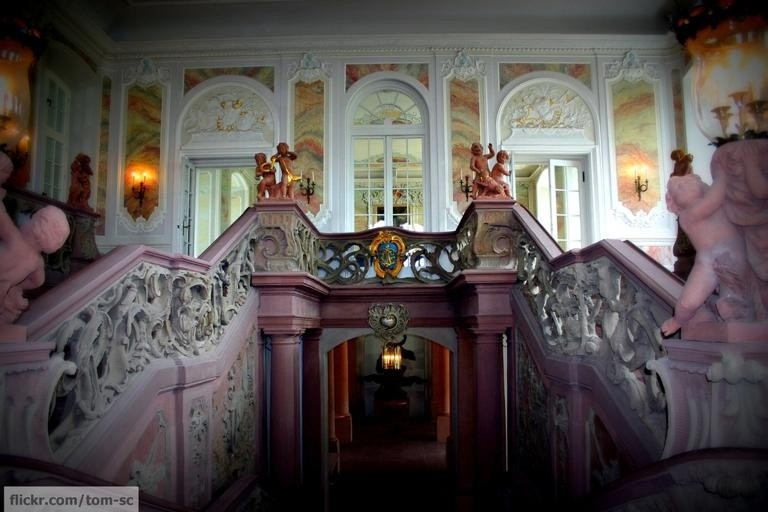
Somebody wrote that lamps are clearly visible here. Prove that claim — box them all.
[299,170,315,204]
[132,173,146,206]
[459,168,474,202]
[634,167,649,201]
[381,342,402,371]
[707,91,768,147]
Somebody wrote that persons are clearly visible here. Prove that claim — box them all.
[475,148,514,200]
[738,140,767,202]
[252,151,276,200]
[269,140,299,199]
[0,151,14,203]
[465,141,495,200]
[137,181,146,208]
[1,196,71,324]
[126,186,139,212]
[471,166,513,200]
[657,165,751,339]
